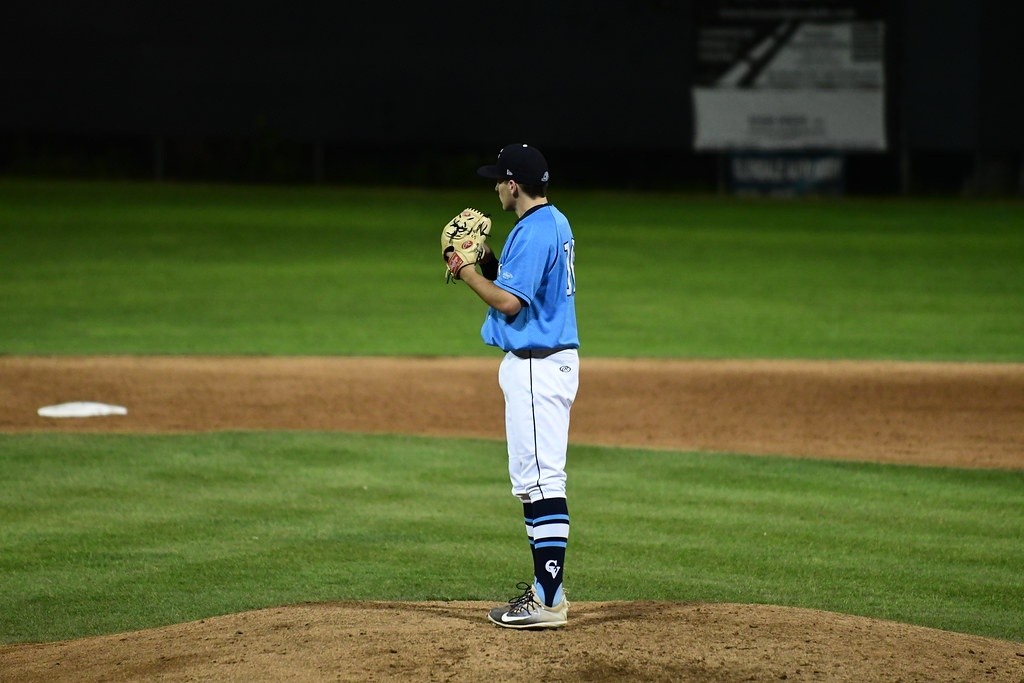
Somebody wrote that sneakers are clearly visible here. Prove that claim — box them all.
[487,582,570,628]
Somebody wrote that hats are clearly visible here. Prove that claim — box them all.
[476,143,550,185]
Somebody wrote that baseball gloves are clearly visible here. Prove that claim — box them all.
[441,209,494,278]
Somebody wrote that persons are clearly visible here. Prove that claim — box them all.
[440,145,579,629]
